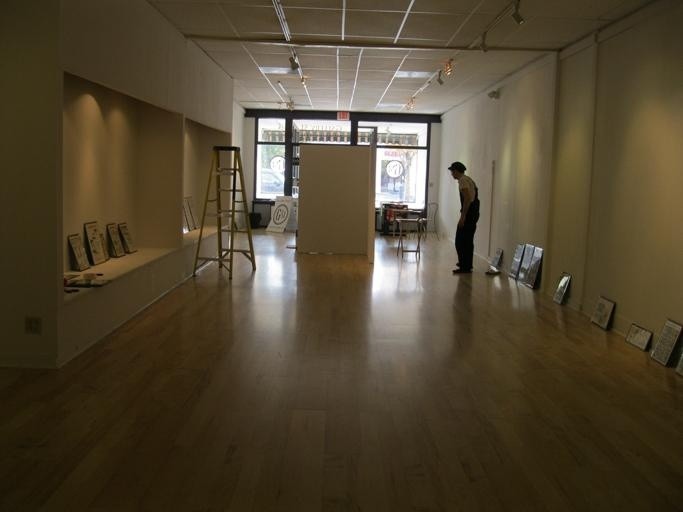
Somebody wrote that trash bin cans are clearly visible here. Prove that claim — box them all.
[249,213,261,229]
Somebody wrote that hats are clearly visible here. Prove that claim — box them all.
[448,162,466,171]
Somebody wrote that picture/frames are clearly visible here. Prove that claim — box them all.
[67,221,137,270]
[591,297,682,379]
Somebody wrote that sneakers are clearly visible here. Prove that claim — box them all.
[453,263,473,273]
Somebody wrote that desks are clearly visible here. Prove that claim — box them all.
[394,217,423,260]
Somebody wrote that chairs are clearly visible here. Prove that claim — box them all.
[418,202,440,241]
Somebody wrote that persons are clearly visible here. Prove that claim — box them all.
[447,162,480,274]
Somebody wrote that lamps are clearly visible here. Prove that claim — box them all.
[466,0,526,53]
[436,70,444,86]
[289,51,299,70]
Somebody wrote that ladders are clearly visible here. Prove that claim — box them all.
[192,145,256,281]
